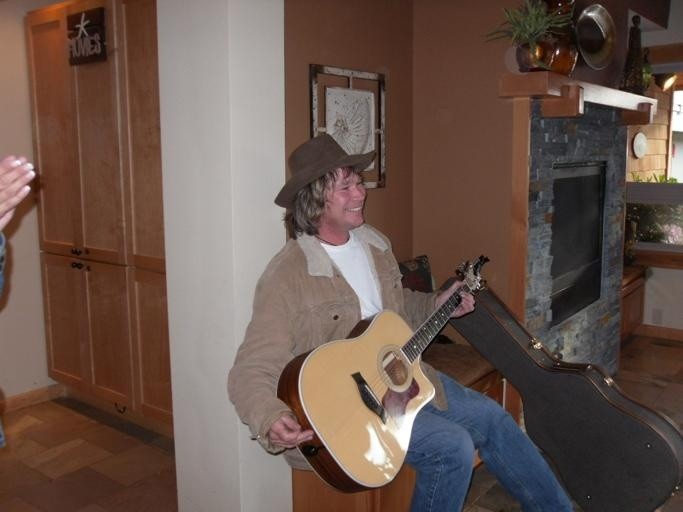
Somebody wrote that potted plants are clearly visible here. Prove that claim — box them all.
[479,0,574,72]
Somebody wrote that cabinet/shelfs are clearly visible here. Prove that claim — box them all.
[622,264,649,343]
[292,340,507,510]
[20,1,174,451]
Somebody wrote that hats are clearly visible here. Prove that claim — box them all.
[275,134,376,210]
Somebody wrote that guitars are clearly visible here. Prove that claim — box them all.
[278,255,489,494]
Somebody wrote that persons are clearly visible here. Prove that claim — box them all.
[1,153,36,296]
[227,132,576,511]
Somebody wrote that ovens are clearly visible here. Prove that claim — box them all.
[551,160,607,328]
